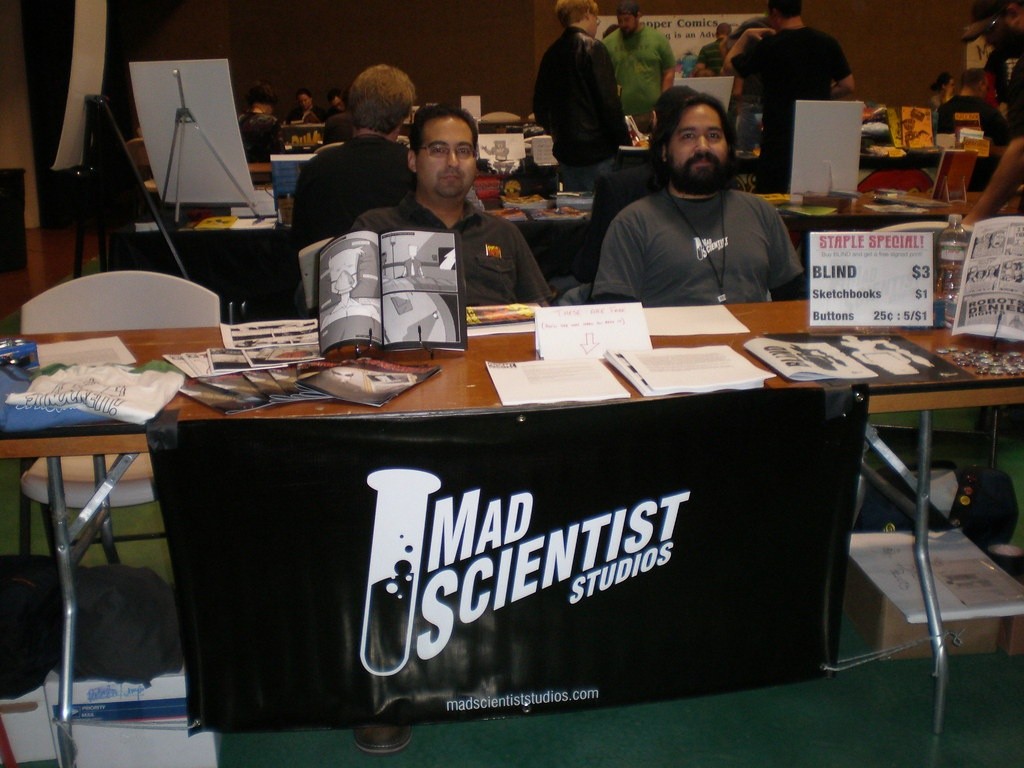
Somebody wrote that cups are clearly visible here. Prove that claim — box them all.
[276,193,295,227]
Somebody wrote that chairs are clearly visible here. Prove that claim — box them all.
[20,270,234,565]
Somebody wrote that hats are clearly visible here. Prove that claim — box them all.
[616,2,638,15]
[960,0,1008,40]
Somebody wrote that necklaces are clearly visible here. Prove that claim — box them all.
[667,189,726,304]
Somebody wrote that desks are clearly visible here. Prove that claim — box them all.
[0,161,1024,754]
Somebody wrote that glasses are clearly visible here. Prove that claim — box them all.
[590,11,601,25]
[417,145,475,159]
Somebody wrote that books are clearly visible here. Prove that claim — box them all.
[614,145,650,171]
[290,110,320,124]
[163,343,442,415]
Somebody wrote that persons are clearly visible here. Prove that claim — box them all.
[577,86,811,310]
[289,63,416,262]
[238,79,282,163]
[286,89,325,122]
[601,2,675,115]
[531,0,632,192]
[927,0,1024,228]
[337,105,553,307]
[693,0,856,193]
[328,88,349,119]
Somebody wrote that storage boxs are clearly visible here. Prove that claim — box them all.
[0,650,221,768]
[843,558,1024,661]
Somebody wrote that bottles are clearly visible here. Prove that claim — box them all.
[934,214,970,328]
